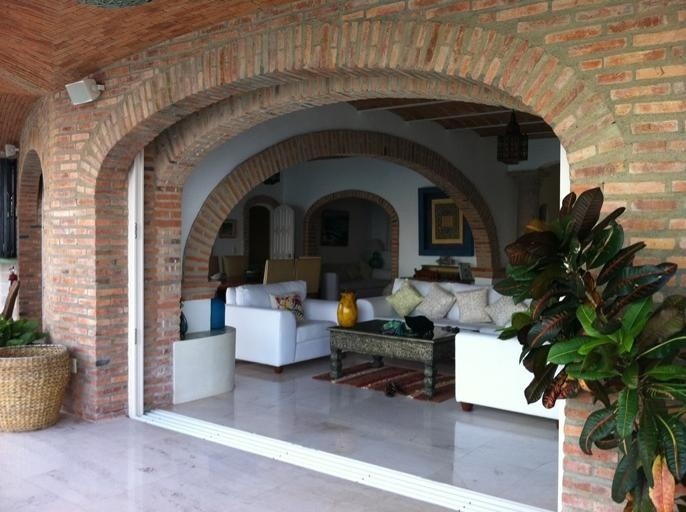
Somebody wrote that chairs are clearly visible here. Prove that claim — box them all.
[454,329,562,429]
[295,256,322,293]
[224,280,340,373]
[0,280,19,320]
[263,260,295,286]
[223,255,248,279]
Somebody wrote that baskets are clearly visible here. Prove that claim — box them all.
[1,343,72,433]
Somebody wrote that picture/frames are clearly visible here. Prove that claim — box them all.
[218,220,236,239]
[431,199,464,245]
[319,208,349,246]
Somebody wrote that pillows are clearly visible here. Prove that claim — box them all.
[384,279,424,319]
[484,295,529,328]
[448,286,492,324]
[416,281,456,319]
[269,291,304,324]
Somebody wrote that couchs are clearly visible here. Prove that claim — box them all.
[355,278,564,333]
[323,262,389,297]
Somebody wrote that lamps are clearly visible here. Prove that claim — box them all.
[497,110,529,165]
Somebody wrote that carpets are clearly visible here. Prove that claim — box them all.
[312,363,455,402]
